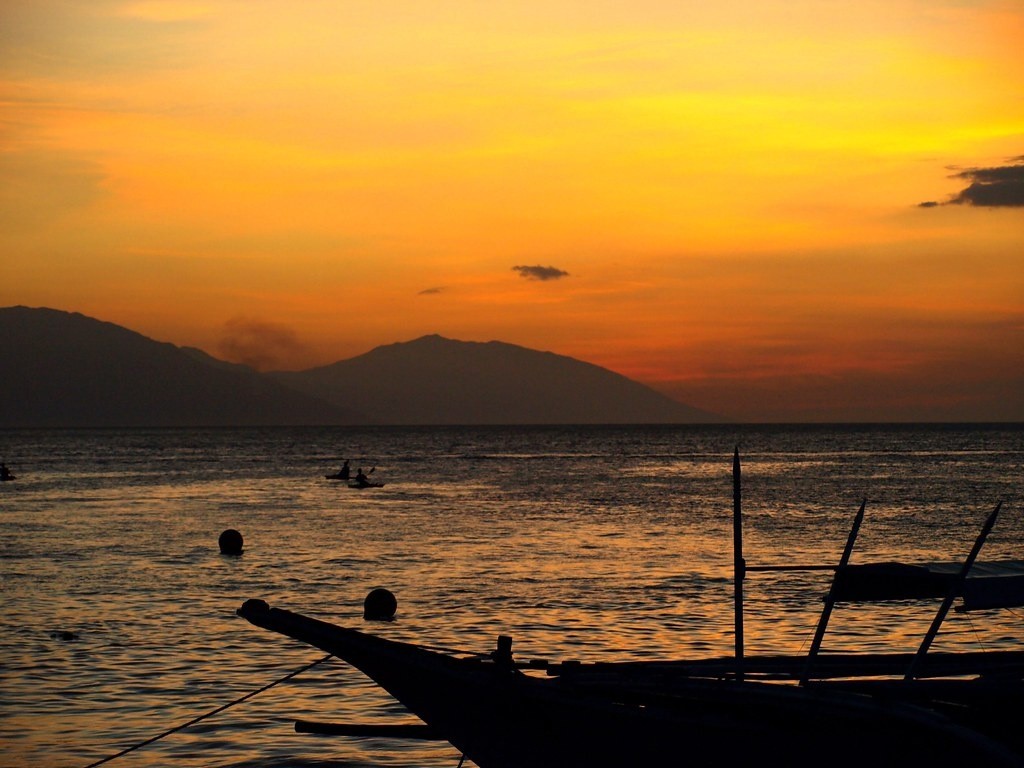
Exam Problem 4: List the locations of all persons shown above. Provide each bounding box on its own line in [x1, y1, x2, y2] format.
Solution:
[337, 461, 350, 478]
[0, 462, 11, 477]
[355, 468, 370, 485]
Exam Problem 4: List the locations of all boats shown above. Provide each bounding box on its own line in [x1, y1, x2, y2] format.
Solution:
[324, 473, 354, 482]
[347, 479, 386, 490]
[231, 446, 1023, 768]
